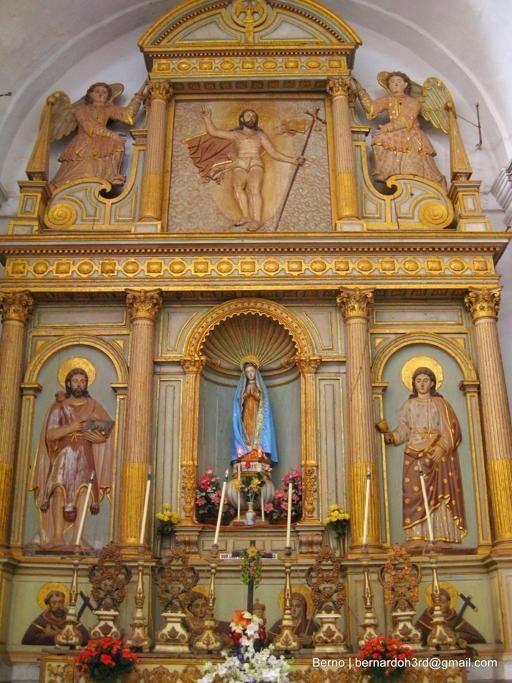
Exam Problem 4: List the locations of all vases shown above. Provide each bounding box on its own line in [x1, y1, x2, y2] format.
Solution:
[245, 502, 257, 525]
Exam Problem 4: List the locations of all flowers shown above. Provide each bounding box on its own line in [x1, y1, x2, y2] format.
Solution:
[156, 502, 181, 536]
[323, 504, 351, 534]
[354, 636, 414, 683]
[195, 465, 237, 525]
[239, 543, 264, 590]
[193, 608, 295, 683]
[263, 464, 305, 524]
[232, 476, 261, 502]
[71, 637, 140, 683]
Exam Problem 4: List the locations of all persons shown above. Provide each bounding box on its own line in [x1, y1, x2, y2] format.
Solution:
[185, 590, 235, 648]
[350, 72, 448, 194]
[198, 104, 306, 233]
[45, 78, 150, 194]
[20, 591, 89, 646]
[384, 367, 468, 542]
[413, 589, 487, 645]
[266, 592, 319, 647]
[42, 368, 107, 518]
[230, 360, 279, 465]
